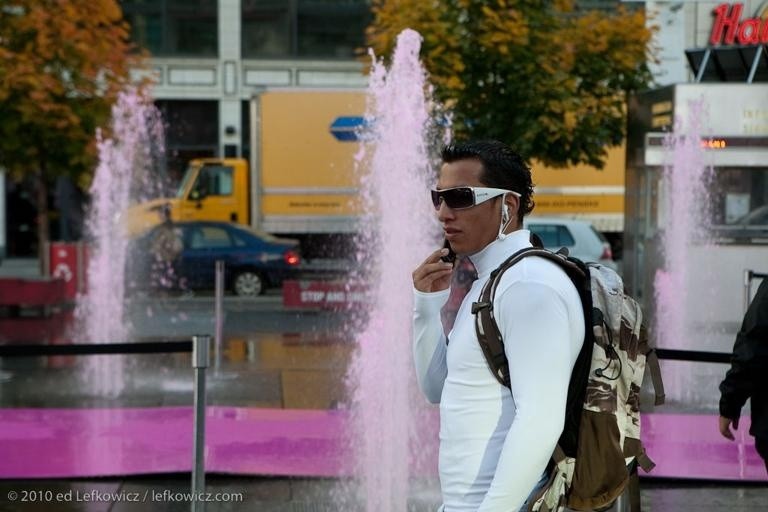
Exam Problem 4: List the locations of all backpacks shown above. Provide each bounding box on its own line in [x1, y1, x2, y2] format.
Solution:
[471, 244, 666, 511]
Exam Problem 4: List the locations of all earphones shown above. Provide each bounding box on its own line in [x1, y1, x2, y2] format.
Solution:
[503, 204, 509, 221]
[499, 224, 505, 240]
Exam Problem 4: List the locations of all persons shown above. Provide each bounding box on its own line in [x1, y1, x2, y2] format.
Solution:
[411, 134, 588, 512]
[717, 272, 768, 477]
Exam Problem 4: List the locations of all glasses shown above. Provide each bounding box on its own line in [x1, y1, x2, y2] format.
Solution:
[430, 186, 522, 211]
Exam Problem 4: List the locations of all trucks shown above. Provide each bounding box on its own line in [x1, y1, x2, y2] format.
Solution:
[124, 89, 627, 262]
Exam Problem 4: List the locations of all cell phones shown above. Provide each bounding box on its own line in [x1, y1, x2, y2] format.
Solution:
[441, 238, 457, 269]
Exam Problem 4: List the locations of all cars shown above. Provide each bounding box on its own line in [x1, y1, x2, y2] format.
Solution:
[124, 220, 302, 297]
[522, 216, 618, 273]
